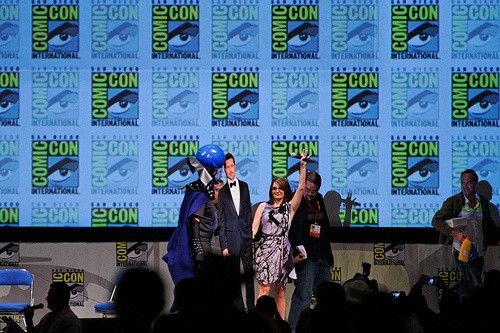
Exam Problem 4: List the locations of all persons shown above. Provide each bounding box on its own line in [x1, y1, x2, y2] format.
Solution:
[163, 144, 225, 314]
[288, 172, 334, 333]
[431, 169, 500, 289]
[1, 263, 500, 333]
[250, 149, 308, 320]
[217, 152, 258, 311]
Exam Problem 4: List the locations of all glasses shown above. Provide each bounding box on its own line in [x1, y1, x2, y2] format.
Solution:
[272, 187, 284, 190]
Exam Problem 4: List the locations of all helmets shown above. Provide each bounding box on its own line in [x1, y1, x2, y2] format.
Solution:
[195, 144, 226, 168]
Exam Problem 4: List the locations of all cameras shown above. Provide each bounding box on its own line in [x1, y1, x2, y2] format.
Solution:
[428, 277, 437, 284]
[391, 292, 400, 297]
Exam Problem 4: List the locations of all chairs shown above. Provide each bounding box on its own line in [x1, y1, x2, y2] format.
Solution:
[94, 271, 124, 318]
[0, 268, 35, 333]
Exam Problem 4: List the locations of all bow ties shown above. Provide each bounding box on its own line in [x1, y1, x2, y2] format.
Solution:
[230, 181, 237, 188]
